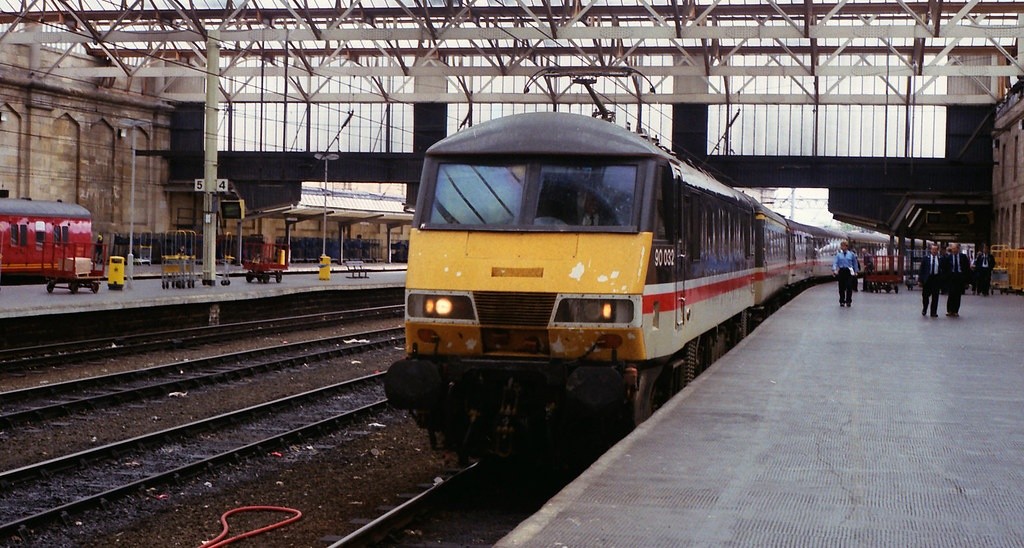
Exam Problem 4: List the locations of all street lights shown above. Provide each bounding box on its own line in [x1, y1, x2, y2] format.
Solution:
[117, 118, 153, 290]
[315, 153, 339, 255]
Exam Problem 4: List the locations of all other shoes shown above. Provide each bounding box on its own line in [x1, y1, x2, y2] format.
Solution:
[945, 311, 952, 316]
[922, 309, 928, 316]
[846, 302, 851, 307]
[840, 302, 846, 307]
[952, 312, 960, 317]
[930, 313, 939, 318]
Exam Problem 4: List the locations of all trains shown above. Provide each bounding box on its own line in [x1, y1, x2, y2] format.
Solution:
[0, 197, 94, 285]
[404, 64, 975, 433]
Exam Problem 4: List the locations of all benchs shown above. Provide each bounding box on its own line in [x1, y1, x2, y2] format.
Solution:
[345, 260, 371, 279]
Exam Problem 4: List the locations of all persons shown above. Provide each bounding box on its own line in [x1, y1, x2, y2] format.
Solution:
[918, 243, 996, 317]
[832, 241, 860, 306]
[577, 194, 613, 226]
[860, 248, 875, 291]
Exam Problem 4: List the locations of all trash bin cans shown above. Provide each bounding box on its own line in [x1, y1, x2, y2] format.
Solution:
[318, 257, 330, 279]
[107, 256, 125, 289]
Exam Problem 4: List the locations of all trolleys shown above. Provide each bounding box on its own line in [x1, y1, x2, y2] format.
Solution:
[161, 230, 196, 289]
[221, 232, 233, 286]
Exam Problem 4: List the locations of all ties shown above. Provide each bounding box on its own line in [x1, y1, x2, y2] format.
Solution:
[954, 254, 963, 274]
[932, 255, 935, 276]
[984, 253, 988, 265]
